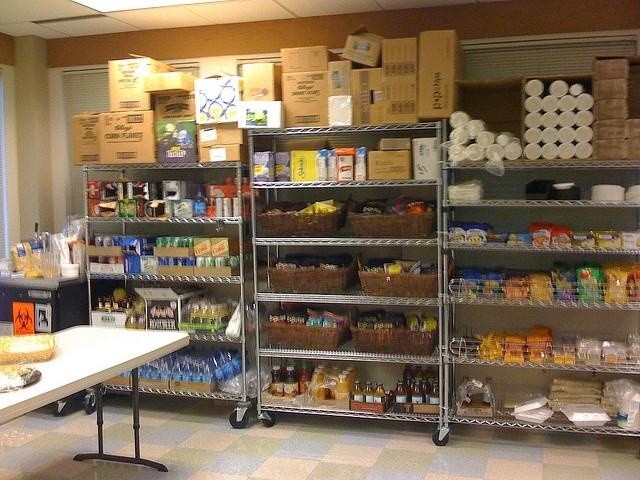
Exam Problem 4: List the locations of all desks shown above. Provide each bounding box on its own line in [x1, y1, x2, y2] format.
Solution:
[0, 322, 191, 472]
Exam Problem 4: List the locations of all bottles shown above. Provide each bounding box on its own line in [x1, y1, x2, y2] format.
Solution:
[268, 359, 441, 414]
[224, 175, 248, 185]
[206, 192, 216, 217]
[480, 376, 495, 406]
[460, 375, 472, 407]
[192, 191, 205, 217]
[94, 291, 239, 335]
[32, 221, 40, 249]
[120, 344, 250, 385]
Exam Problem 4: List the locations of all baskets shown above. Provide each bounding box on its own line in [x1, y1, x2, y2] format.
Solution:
[266, 255, 356, 292]
[0, 332, 57, 364]
[258, 198, 348, 239]
[262, 309, 349, 350]
[357, 254, 438, 297]
[346, 198, 437, 239]
[348, 312, 439, 356]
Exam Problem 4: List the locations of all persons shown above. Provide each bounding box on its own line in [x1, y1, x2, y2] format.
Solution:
[38, 310, 49, 327]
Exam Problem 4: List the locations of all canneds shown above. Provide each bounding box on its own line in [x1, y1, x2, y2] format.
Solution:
[95, 236, 239, 267]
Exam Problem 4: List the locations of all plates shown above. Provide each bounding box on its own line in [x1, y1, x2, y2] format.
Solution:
[626, 185, 640, 203]
[552, 181, 576, 191]
[590, 183, 625, 202]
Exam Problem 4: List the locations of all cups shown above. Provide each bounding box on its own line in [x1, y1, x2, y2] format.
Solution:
[32, 250, 62, 280]
